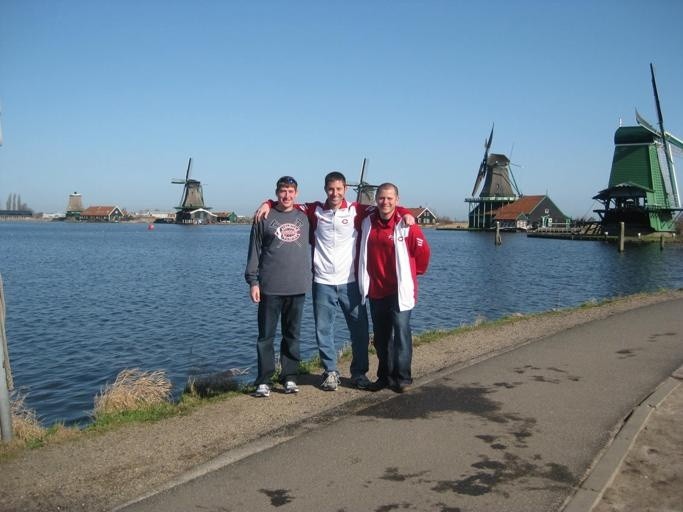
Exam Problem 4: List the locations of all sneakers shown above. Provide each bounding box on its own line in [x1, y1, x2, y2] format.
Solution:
[320, 371, 340, 391]
[255, 382, 274, 397]
[282, 380, 299, 394]
[367, 375, 395, 392]
[350, 374, 372, 390]
[393, 379, 417, 393]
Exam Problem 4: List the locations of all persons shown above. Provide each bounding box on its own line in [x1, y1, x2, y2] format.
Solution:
[353, 184, 429, 393]
[253, 171, 416, 391]
[242, 175, 311, 397]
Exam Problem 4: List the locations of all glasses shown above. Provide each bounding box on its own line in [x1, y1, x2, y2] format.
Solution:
[277, 178, 297, 185]
